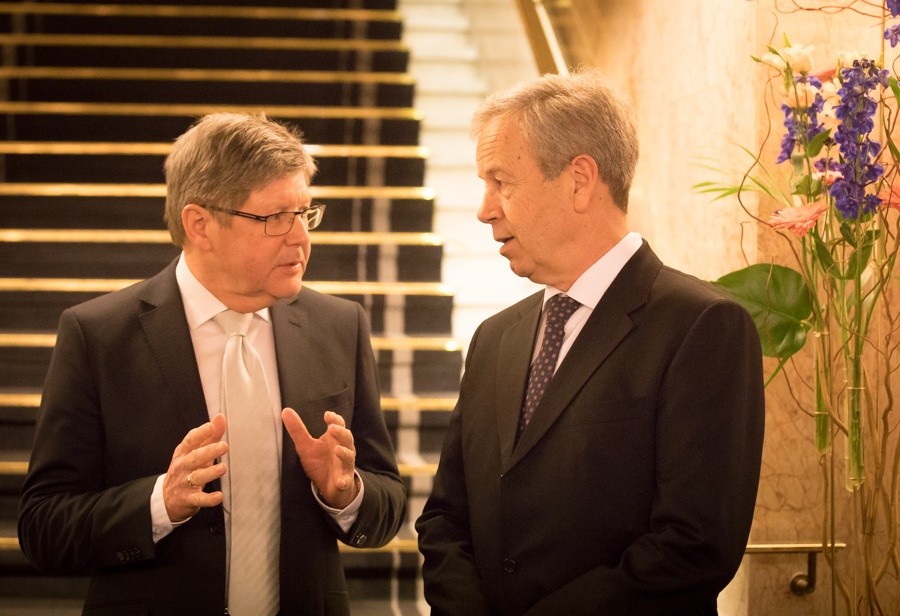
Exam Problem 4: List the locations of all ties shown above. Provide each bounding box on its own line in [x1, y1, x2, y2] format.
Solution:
[515, 295, 582, 446]
[214, 310, 282, 616]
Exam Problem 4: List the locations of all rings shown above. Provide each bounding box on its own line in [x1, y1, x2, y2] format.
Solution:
[187, 473, 194, 486]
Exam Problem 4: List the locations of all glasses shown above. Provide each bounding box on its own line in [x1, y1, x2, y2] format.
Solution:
[199, 202, 326, 236]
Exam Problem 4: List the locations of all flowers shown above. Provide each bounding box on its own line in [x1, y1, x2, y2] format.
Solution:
[691, 0, 900, 616]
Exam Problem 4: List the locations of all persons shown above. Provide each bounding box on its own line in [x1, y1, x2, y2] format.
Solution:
[415, 70, 767, 616]
[16, 113, 407, 616]
